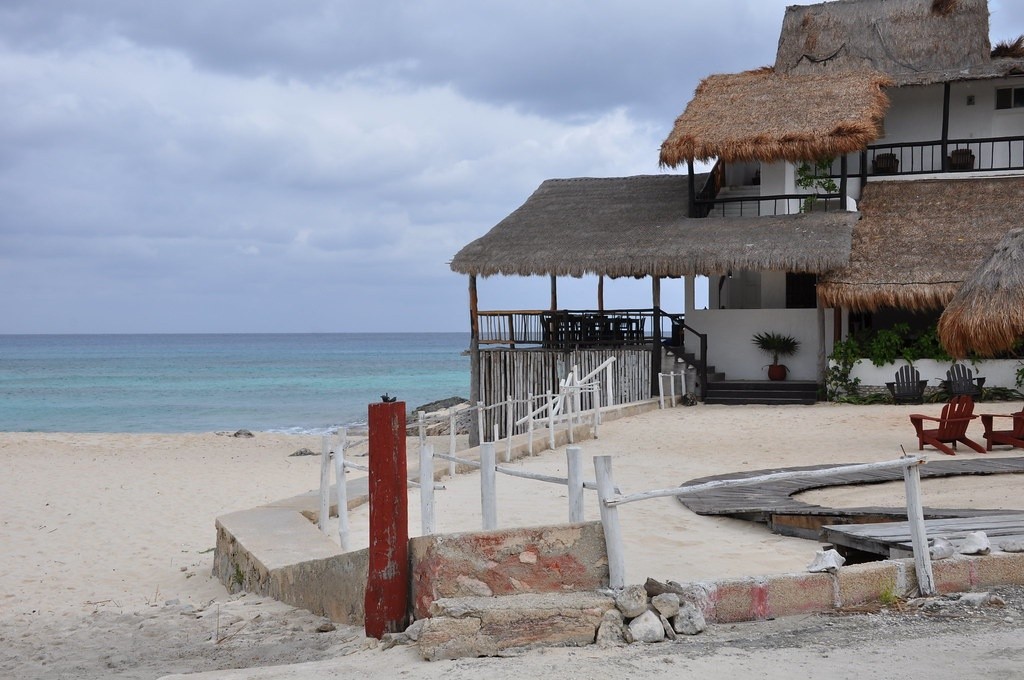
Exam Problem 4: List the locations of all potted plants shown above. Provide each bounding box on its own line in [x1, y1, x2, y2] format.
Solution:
[752, 332, 802, 382]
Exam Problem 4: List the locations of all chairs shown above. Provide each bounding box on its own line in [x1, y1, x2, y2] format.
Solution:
[910, 394, 988, 456]
[939, 364, 986, 404]
[872, 152, 899, 175]
[947, 149, 973, 170]
[885, 365, 929, 405]
[539, 309, 646, 349]
[980, 406, 1024, 452]
